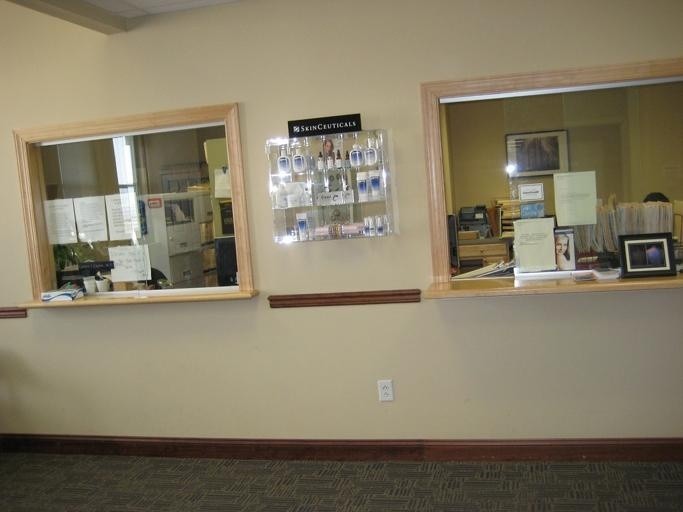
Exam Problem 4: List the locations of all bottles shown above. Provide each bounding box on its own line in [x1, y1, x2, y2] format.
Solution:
[317, 150, 350, 168]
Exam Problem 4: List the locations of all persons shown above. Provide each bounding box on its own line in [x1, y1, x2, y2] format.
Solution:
[554, 232, 574, 270]
[317, 139, 336, 163]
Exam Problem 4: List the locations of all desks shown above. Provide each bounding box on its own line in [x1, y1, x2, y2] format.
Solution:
[457, 240, 510, 273]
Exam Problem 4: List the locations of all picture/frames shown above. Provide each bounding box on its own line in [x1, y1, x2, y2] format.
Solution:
[505, 129, 570, 181]
[616, 231, 677, 279]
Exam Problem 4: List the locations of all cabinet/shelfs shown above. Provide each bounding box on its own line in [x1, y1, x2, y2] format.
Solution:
[265, 124, 398, 247]
[143, 193, 204, 289]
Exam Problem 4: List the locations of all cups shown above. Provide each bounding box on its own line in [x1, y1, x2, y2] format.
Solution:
[96, 277, 113, 292]
[84, 276, 97, 293]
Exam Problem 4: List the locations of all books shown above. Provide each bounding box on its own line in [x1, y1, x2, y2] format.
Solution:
[486, 208, 502, 237]
[568, 192, 674, 254]
[450, 262, 500, 279]
[496, 199, 521, 240]
